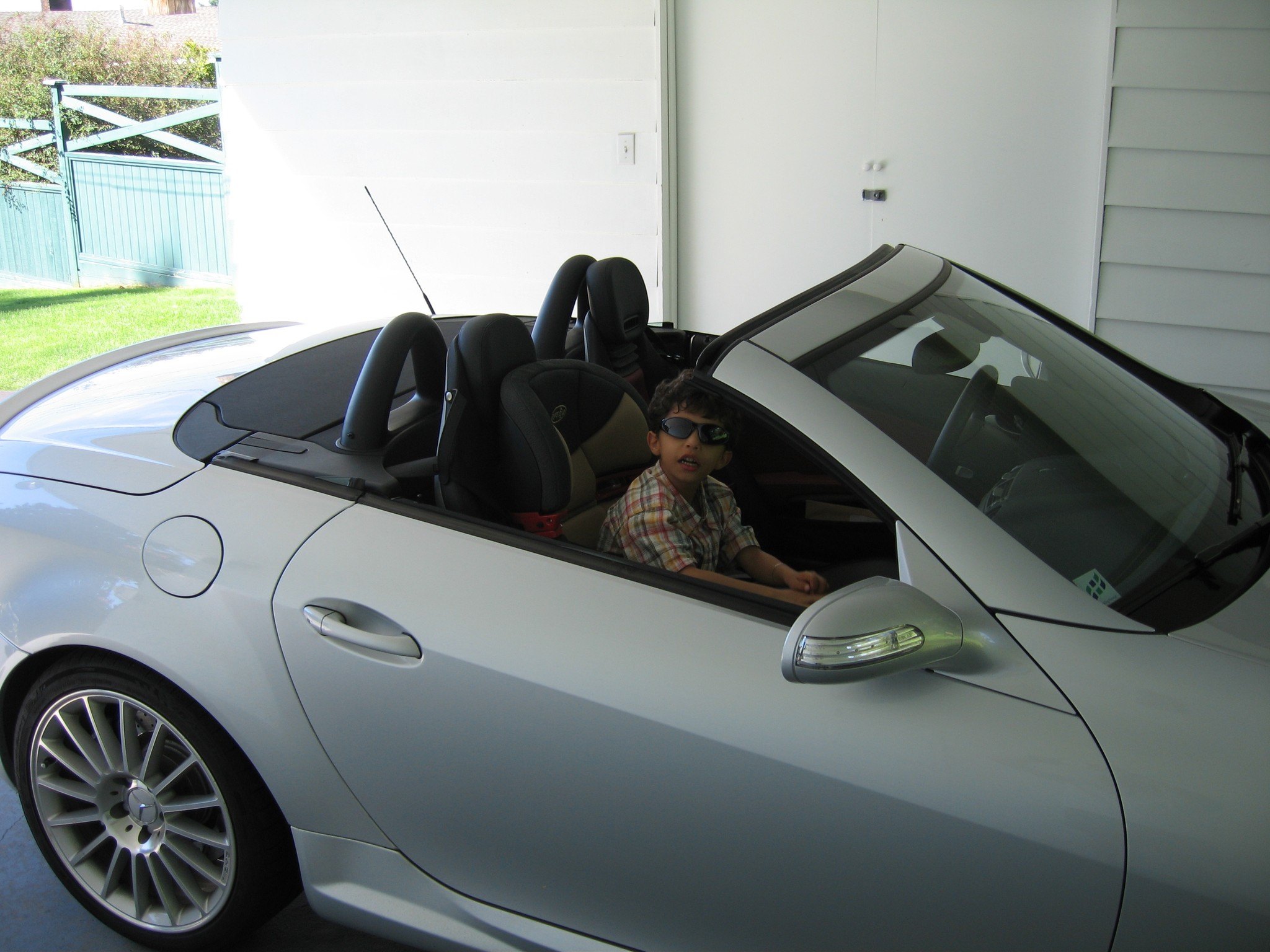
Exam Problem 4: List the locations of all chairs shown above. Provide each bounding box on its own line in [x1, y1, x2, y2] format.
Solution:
[434, 256, 659, 549]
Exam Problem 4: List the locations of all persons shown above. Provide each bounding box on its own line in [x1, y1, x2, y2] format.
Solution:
[596, 368, 829, 605]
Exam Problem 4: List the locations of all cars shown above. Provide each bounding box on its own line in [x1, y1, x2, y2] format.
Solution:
[0, 240, 1270, 952]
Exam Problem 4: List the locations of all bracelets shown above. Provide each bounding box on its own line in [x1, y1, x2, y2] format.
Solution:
[771, 563, 786, 584]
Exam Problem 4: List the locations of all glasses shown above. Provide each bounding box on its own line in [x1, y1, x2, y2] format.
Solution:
[651, 417, 728, 449]
